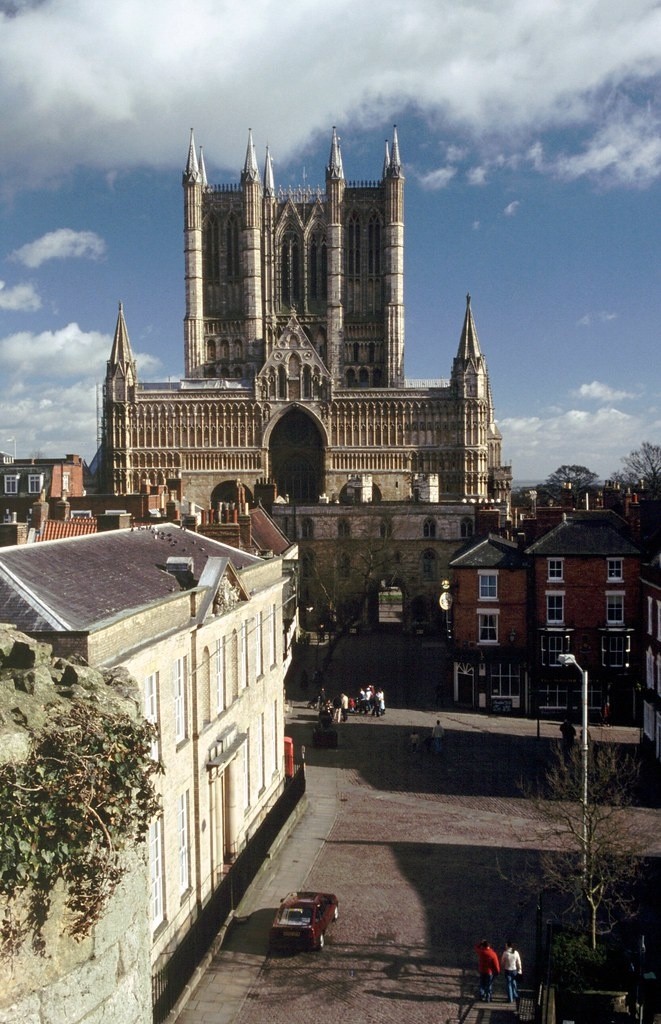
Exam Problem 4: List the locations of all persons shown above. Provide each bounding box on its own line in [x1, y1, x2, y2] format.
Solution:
[502, 944, 522, 1002]
[474, 937, 501, 1003]
[316, 684, 385, 723]
[559, 717, 576, 758]
[433, 719, 444, 752]
[409, 731, 420, 753]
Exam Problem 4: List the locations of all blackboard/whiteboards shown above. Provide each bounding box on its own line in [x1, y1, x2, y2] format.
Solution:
[492, 698, 512, 714]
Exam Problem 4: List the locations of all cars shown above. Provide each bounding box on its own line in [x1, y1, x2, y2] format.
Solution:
[268, 890, 339, 950]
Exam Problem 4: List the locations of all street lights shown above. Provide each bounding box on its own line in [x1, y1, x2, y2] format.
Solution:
[559, 654, 587, 877]
